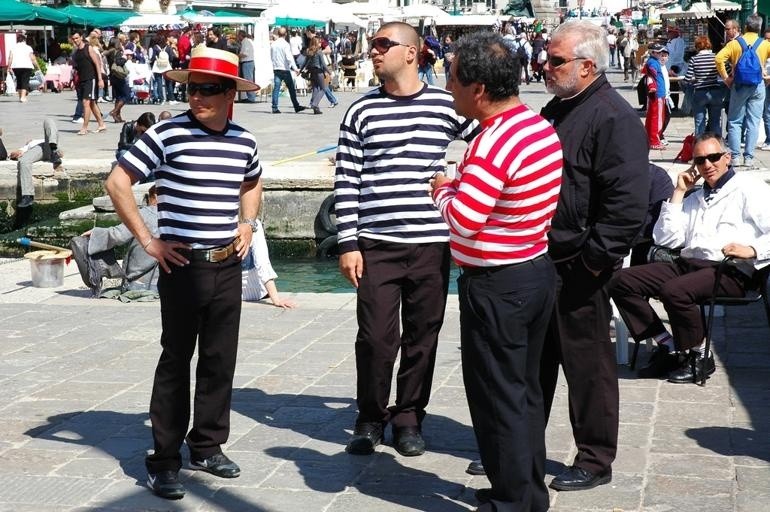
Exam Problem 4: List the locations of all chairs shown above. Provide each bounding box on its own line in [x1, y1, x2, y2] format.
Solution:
[272, 60, 374, 106]
[29, 60, 188, 105]
[626, 244, 770, 389]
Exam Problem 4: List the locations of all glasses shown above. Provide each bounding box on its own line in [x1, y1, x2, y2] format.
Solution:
[186, 82, 230, 96]
[546, 53, 597, 69]
[693, 152, 727, 165]
[367, 37, 410, 55]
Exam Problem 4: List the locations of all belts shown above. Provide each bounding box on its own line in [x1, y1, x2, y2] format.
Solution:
[173, 238, 241, 264]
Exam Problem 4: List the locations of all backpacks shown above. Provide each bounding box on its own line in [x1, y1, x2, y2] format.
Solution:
[154, 45, 169, 67]
[516, 41, 528, 65]
[734, 35, 765, 90]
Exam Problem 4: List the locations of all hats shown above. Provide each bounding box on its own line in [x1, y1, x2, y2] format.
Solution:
[648, 43, 669, 56]
[163, 46, 260, 92]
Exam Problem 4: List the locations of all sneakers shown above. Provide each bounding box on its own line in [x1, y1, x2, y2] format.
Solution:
[345, 421, 386, 455]
[147, 470, 185, 497]
[188, 452, 240, 478]
[271, 100, 338, 115]
[54, 154, 62, 164]
[72, 111, 125, 135]
[649, 138, 668, 150]
[18, 195, 34, 208]
[393, 430, 426, 456]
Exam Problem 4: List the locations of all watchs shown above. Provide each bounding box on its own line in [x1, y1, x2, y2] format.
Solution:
[238, 218, 259, 232]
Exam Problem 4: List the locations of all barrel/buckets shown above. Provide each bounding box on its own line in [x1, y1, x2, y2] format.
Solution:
[24, 250, 72, 288]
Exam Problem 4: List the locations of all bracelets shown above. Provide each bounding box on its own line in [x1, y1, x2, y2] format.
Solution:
[142, 234, 153, 250]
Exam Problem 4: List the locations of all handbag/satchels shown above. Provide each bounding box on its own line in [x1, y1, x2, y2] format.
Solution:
[111, 62, 130, 80]
[537, 50, 548, 65]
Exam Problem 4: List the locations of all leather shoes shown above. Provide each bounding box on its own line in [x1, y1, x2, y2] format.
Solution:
[550, 466, 612, 491]
[637, 344, 689, 379]
[668, 350, 716, 384]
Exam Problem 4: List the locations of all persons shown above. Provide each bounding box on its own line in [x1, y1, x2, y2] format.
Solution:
[333, 21, 480, 458]
[466, 19, 650, 490]
[611, 132, 769, 384]
[103, 46, 262, 499]
[79, 184, 163, 300]
[241, 219, 293, 310]
[428, 33, 565, 511]
